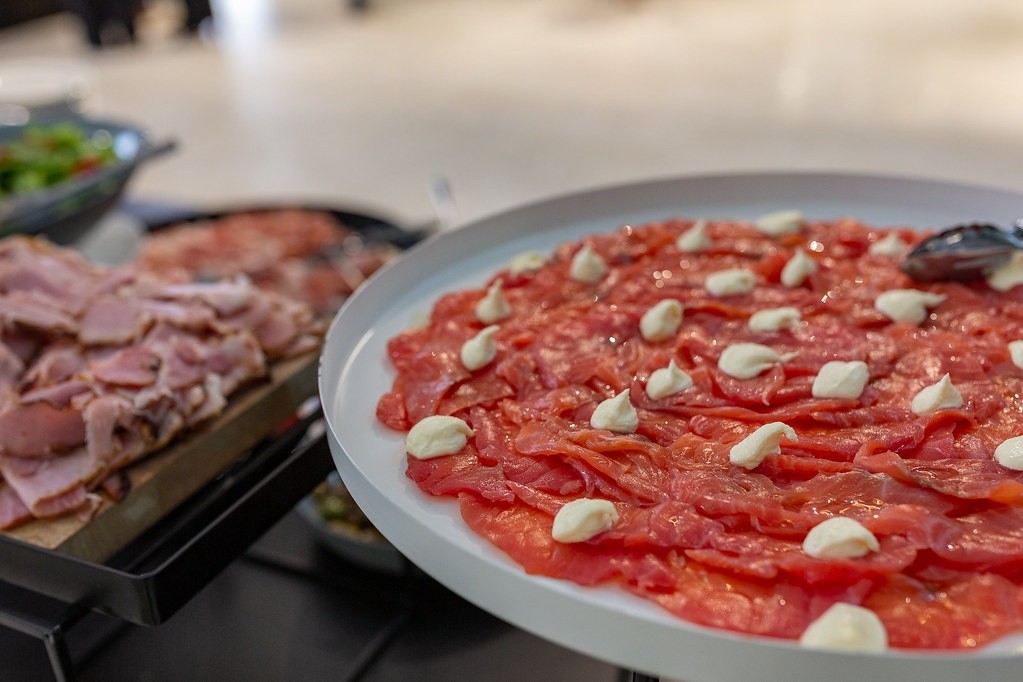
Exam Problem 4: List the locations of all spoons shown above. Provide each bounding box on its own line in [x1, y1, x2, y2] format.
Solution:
[901, 225, 1022, 281]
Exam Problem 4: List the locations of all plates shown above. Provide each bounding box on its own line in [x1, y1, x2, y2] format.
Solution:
[1, 102, 181, 234]
[1, 203, 436, 629]
[317, 169, 1023, 682]
